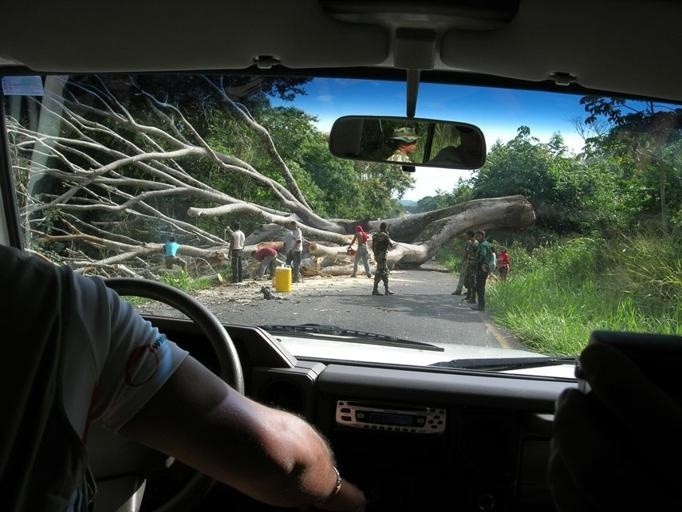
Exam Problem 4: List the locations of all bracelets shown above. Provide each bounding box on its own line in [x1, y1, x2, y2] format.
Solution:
[332, 467, 342, 496]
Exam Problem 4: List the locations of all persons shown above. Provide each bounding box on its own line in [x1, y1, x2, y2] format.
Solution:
[470, 229, 492, 310]
[250, 248, 278, 281]
[2, 241, 368, 512]
[349, 225, 372, 278]
[282, 223, 293, 254]
[498, 245, 511, 284]
[370, 222, 397, 296]
[163, 236, 188, 275]
[225, 222, 246, 284]
[451, 230, 479, 300]
[430, 124, 483, 166]
[383, 126, 422, 164]
[489, 247, 499, 282]
[287, 220, 303, 285]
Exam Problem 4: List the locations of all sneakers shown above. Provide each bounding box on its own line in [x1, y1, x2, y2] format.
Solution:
[450, 290, 486, 311]
[350, 273, 372, 278]
[371, 291, 395, 296]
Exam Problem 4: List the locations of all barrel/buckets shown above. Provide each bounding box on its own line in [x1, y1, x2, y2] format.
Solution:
[275, 263, 292, 291]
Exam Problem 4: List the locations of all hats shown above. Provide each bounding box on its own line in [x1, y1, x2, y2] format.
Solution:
[390, 127, 423, 145]
